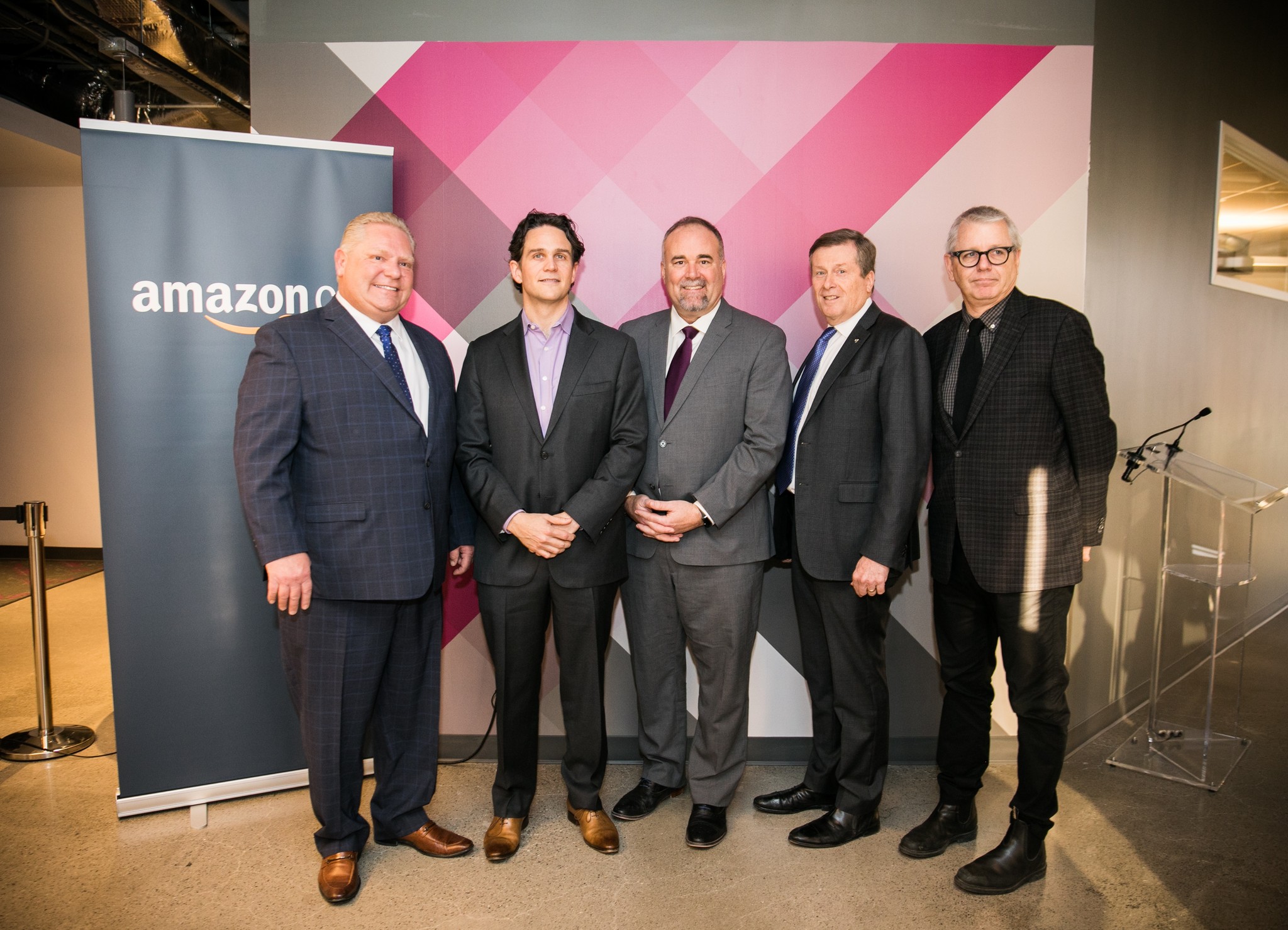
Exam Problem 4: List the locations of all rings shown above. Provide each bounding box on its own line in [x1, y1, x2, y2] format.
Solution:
[867, 587, 876, 593]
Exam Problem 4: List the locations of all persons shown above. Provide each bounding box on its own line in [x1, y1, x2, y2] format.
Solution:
[748, 228, 936, 848]
[895, 204, 1121, 899]
[229, 208, 477, 904]
[599, 213, 793, 851]
[451, 204, 651, 865]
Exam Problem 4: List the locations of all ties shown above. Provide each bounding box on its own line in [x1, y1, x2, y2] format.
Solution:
[952, 318, 986, 442]
[664, 326, 699, 423]
[375, 325, 414, 412]
[773, 326, 836, 496]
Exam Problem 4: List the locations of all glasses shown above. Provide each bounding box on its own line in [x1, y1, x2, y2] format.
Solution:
[947, 245, 1016, 268]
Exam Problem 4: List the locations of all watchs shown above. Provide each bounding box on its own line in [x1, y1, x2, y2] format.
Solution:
[696, 506, 713, 528]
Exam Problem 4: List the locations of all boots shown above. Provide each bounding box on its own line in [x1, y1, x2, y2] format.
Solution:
[954, 805, 1047, 894]
[898, 796, 978, 857]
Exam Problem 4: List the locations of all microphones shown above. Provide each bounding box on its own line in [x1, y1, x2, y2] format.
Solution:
[1126, 407, 1212, 462]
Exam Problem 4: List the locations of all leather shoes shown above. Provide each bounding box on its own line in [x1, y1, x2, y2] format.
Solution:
[567, 796, 620, 853]
[788, 802, 881, 849]
[374, 819, 475, 858]
[685, 802, 728, 848]
[753, 782, 837, 814]
[318, 851, 362, 902]
[611, 777, 688, 821]
[484, 812, 529, 861]
